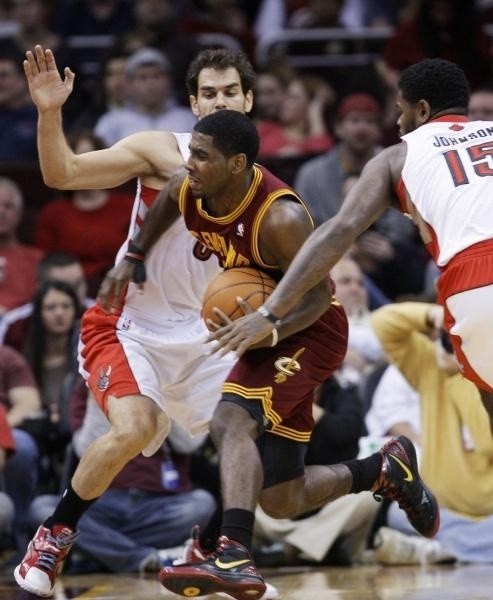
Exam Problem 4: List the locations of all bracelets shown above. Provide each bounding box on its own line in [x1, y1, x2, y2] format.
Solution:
[124, 251, 145, 262]
[257, 304, 282, 325]
[271, 328, 278, 347]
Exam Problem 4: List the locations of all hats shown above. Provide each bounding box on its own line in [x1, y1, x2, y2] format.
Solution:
[126, 47, 170, 75]
[338, 93, 379, 119]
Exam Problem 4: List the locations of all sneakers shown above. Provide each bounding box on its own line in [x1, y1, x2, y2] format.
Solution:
[155, 545, 184, 568]
[159, 537, 266, 600]
[374, 527, 439, 567]
[172, 524, 278, 600]
[372, 434, 440, 537]
[12, 523, 81, 597]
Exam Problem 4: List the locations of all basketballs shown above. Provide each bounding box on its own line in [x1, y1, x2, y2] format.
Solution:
[202, 267, 278, 333]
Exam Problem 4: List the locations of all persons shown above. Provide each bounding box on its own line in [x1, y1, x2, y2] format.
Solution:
[0, 0, 68, 57]
[99, 110, 443, 600]
[0, 178, 43, 312]
[12, 45, 254, 596]
[370, 302, 493, 563]
[94, 48, 197, 148]
[0, 252, 95, 351]
[250, 70, 285, 135]
[0, 35, 69, 170]
[21, 282, 84, 440]
[259, 76, 333, 158]
[202, 57, 493, 392]
[0, 345, 42, 522]
[37, 129, 135, 299]
[296, 94, 417, 273]
[87, 51, 134, 124]
[467, 90, 492, 122]
[26, 443, 217, 575]
[327, 259, 390, 413]
[364, 361, 421, 467]
